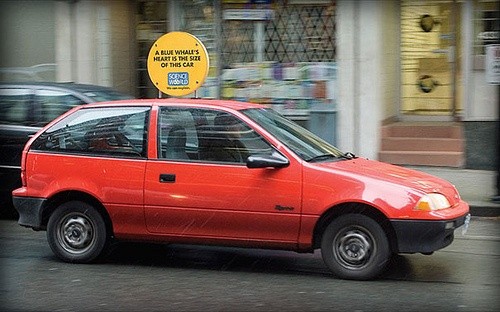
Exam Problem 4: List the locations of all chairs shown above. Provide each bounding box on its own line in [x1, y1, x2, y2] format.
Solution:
[166, 127, 191, 160]
[206, 112, 229, 161]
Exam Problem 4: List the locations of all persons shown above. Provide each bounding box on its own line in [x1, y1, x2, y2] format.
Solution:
[206, 112, 252, 163]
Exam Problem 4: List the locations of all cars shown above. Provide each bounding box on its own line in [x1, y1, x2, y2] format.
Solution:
[12, 99, 471, 280]
[0, 83, 135, 186]
